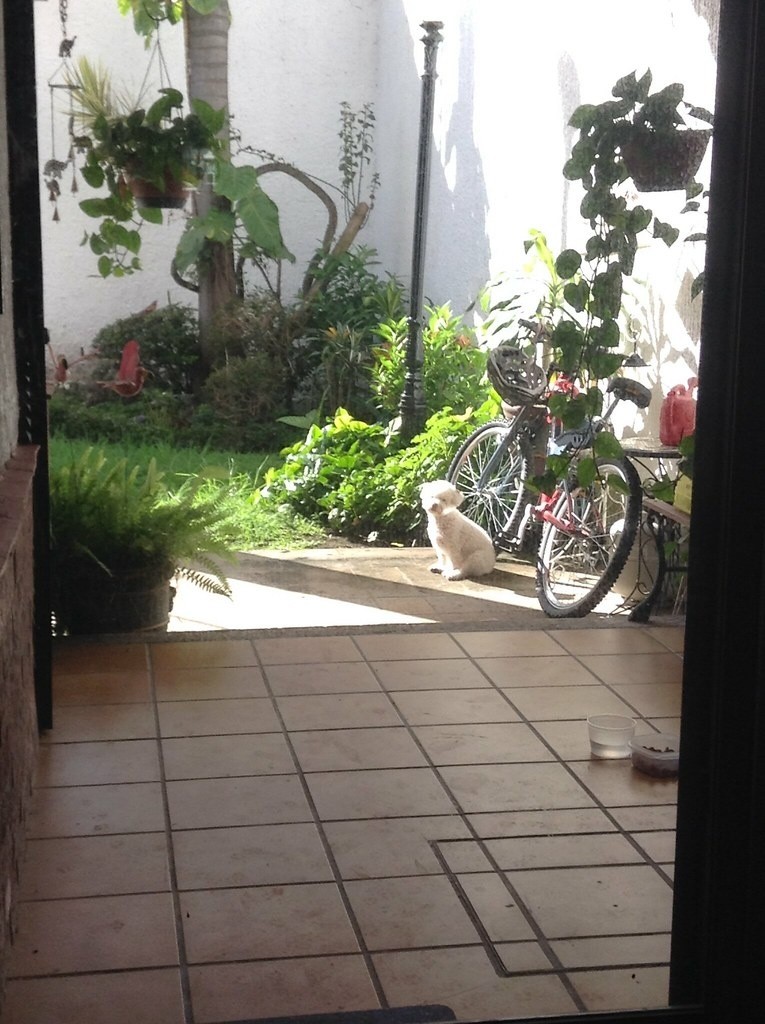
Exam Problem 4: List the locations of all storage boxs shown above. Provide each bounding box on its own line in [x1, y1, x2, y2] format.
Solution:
[627, 734, 679, 777]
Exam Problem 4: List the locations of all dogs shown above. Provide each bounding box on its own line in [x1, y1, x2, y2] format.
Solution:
[416, 479, 496, 582]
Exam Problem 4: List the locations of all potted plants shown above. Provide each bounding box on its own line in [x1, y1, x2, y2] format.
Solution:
[522, 66, 713, 503]
[47, 445, 241, 632]
[66, 89, 230, 279]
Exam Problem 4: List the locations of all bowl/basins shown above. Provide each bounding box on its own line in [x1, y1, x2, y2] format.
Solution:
[587, 713, 637, 759]
[627, 733, 679, 777]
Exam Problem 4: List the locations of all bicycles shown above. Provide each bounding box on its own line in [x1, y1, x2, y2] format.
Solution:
[443, 318, 644, 620]
